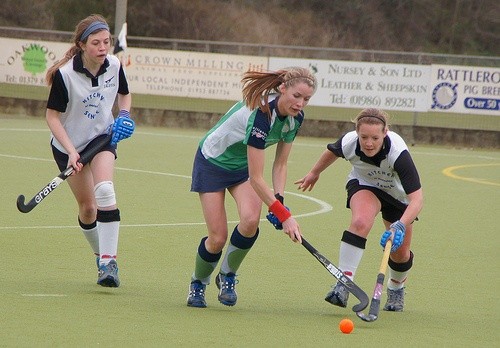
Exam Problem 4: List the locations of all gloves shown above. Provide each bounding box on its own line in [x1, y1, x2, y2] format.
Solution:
[266, 194, 289, 230]
[109, 110, 135, 146]
[381, 221, 406, 252]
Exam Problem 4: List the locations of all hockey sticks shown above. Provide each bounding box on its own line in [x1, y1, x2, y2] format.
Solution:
[16, 135, 112, 214]
[294, 234, 369, 312]
[356, 228, 396, 322]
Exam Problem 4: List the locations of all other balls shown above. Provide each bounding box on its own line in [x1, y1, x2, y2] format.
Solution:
[339, 319, 354, 334]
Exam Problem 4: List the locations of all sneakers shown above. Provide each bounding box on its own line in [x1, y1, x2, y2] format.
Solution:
[325, 282, 351, 308]
[383, 286, 407, 311]
[186, 280, 207, 308]
[215, 272, 240, 307]
[94, 254, 121, 288]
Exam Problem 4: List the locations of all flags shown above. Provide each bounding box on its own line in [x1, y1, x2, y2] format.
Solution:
[113, 23, 128, 54]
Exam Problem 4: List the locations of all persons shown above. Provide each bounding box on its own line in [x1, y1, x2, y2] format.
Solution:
[186, 67, 317, 309]
[294, 107, 424, 312]
[45, 15, 135, 286]
